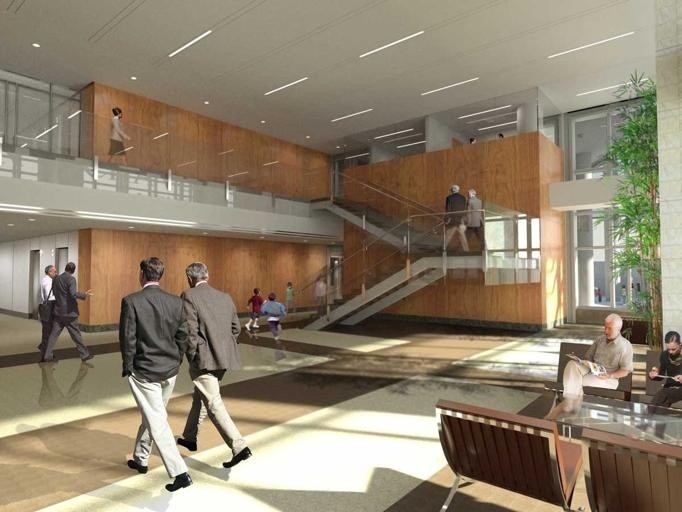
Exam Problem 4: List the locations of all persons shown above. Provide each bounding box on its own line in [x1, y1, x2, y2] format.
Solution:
[285, 282, 297, 316]
[109, 108, 131, 170]
[38, 265, 58, 360]
[595, 281, 640, 303]
[179, 261, 253, 470]
[244, 287, 265, 330]
[442, 185, 470, 254]
[315, 275, 326, 318]
[41, 262, 95, 362]
[117, 256, 194, 492]
[561, 312, 634, 416]
[262, 293, 286, 341]
[648, 330, 682, 408]
[458, 189, 484, 252]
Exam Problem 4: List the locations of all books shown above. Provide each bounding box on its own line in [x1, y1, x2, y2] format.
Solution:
[565, 352, 605, 376]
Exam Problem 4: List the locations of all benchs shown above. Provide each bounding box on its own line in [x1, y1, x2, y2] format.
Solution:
[580, 427, 681, 511]
[435, 398, 587, 511]
[638, 350, 681, 409]
[544, 341, 632, 406]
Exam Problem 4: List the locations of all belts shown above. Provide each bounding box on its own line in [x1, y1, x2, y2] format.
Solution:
[43, 301, 56, 303]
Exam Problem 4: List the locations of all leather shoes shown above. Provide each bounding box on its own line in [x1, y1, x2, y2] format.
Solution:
[178, 438, 197, 451]
[223, 447, 252, 468]
[128, 460, 147, 473]
[39, 344, 56, 361]
[166, 472, 193, 491]
[82, 356, 94, 361]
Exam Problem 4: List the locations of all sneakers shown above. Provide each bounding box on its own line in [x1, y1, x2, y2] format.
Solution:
[253, 324, 259, 328]
[245, 324, 251, 331]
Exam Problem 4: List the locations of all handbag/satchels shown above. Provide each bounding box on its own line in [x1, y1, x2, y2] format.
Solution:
[39, 303, 52, 322]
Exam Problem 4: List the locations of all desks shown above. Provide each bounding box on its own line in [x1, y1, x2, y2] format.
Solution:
[543, 394, 682, 461]
